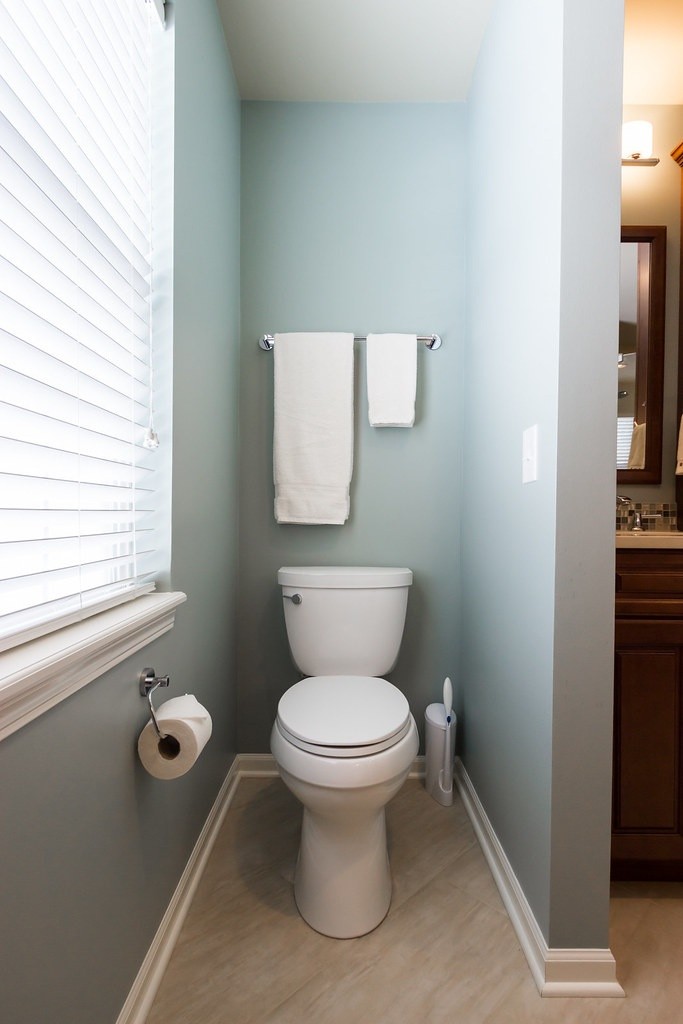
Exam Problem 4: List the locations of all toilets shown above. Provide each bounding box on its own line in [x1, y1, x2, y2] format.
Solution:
[270, 566, 420, 940]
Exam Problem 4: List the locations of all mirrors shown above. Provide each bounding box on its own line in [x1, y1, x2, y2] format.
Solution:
[617, 222, 666, 488]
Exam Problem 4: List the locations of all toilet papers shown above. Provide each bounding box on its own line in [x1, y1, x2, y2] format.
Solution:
[138, 693, 213, 779]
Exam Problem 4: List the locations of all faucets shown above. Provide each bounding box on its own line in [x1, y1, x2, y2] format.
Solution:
[616, 493, 633, 512]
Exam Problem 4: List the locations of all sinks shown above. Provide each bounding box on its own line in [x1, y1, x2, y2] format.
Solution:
[616, 533, 636, 536]
[638, 533, 683, 536]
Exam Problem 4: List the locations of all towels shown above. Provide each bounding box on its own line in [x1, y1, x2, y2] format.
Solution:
[273, 332, 355, 525]
[628, 422, 647, 470]
[675, 413, 683, 475]
[366, 332, 418, 428]
